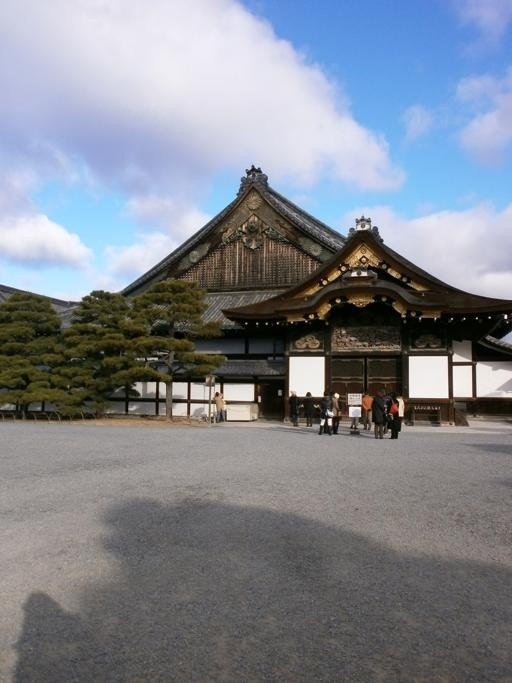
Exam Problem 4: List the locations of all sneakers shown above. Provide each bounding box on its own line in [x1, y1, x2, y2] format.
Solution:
[318, 425, 339, 435]
[350, 425, 401, 440]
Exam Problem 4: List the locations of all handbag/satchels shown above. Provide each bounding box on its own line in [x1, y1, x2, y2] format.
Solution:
[390, 403, 399, 415]
[325, 409, 335, 419]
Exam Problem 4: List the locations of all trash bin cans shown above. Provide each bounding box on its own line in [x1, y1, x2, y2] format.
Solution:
[455, 401, 467, 426]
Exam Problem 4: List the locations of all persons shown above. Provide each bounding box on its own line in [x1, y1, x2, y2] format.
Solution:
[288, 389, 408, 439]
[214, 392, 228, 422]
